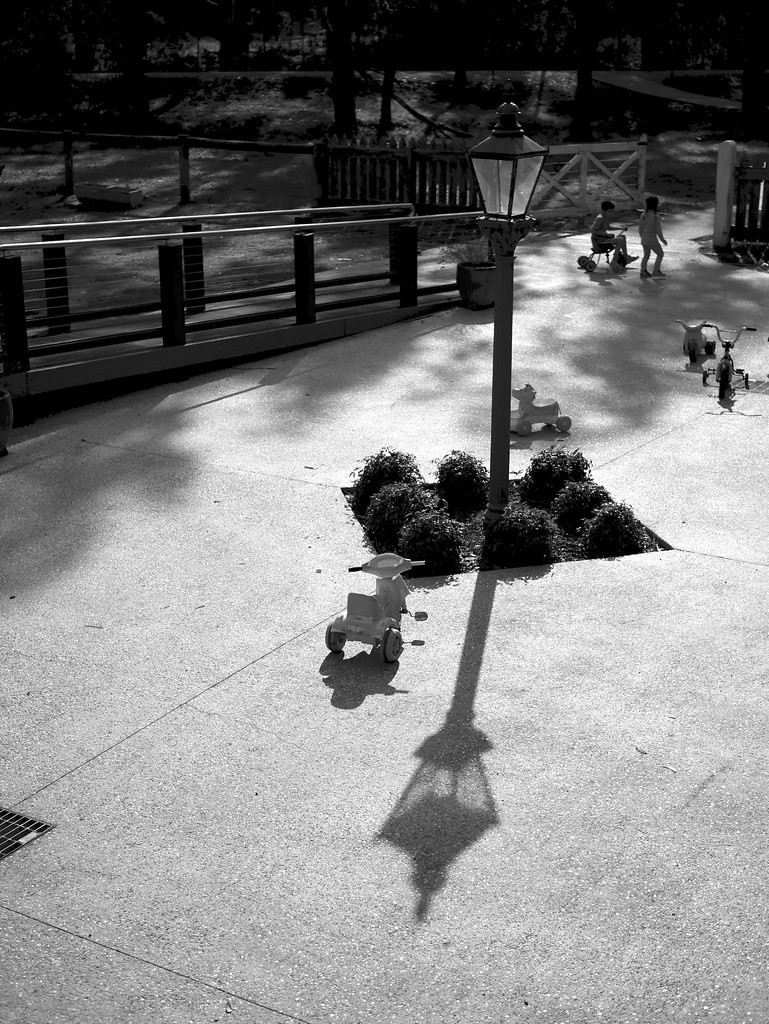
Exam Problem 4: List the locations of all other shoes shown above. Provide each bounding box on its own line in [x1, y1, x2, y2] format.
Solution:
[624, 255, 639, 265]
[609, 261, 625, 270]
[650, 270, 665, 277]
[640, 271, 652, 278]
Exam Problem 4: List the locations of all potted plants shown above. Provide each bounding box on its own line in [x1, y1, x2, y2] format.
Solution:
[437, 241, 495, 312]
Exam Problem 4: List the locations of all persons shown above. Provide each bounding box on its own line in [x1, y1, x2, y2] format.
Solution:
[589, 201, 639, 271]
[639, 196, 668, 278]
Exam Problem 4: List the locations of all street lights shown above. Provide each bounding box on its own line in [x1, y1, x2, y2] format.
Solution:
[461, 75, 552, 524]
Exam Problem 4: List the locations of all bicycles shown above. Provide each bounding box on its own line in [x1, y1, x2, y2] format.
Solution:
[701, 321, 757, 404]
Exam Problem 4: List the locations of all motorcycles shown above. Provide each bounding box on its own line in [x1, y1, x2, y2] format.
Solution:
[675, 319, 716, 363]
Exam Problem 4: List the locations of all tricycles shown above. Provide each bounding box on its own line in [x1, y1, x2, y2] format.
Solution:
[577, 226, 630, 273]
[322, 553, 429, 662]
